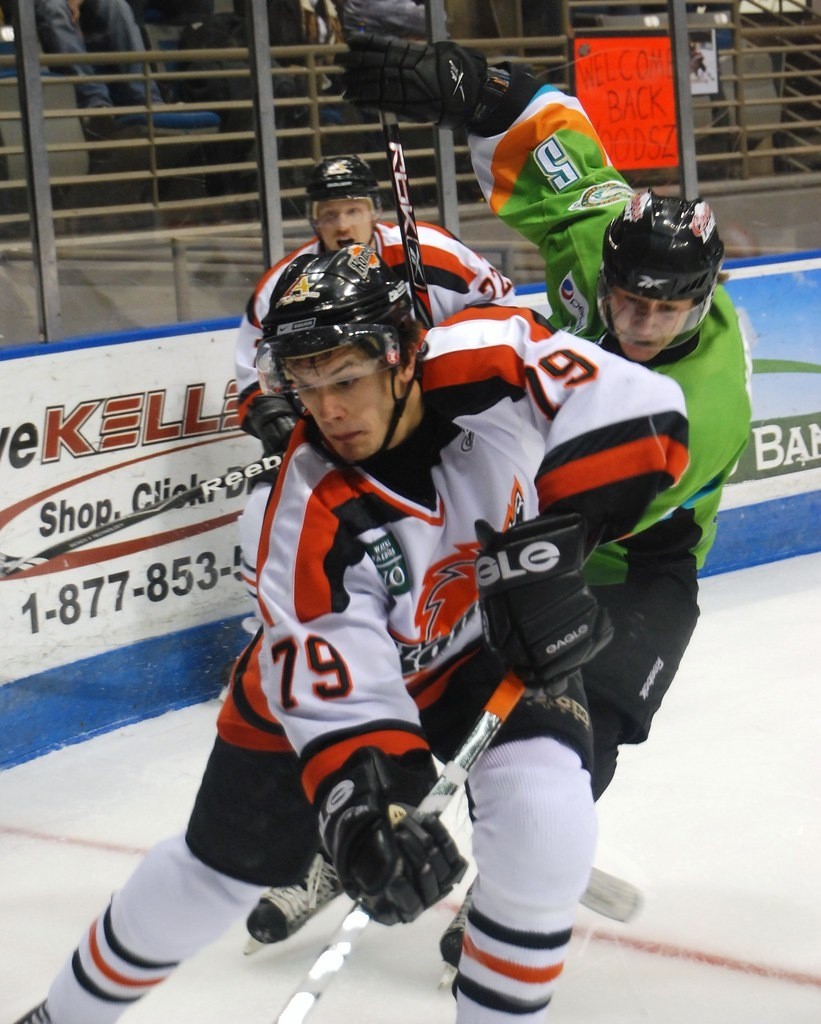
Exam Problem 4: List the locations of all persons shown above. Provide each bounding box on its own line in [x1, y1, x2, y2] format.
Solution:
[243, 28, 753, 975]
[9, 239, 694, 1024]
[1, 0, 444, 217]
[233, 150, 520, 473]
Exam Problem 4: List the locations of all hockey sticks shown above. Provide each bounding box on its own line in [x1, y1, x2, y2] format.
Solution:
[366, 104, 644, 922]
[269, 677, 529, 1024]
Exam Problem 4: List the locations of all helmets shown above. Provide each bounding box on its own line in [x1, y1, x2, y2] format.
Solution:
[596, 188, 724, 350]
[255, 242, 414, 394]
[304, 156, 382, 228]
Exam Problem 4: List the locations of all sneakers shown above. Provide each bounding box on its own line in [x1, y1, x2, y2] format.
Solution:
[245, 855, 344, 958]
[438, 873, 478, 987]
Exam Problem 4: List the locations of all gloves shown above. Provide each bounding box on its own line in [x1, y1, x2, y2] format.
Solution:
[315, 745, 468, 924]
[474, 514, 614, 702]
[246, 400, 297, 479]
[334, 35, 488, 130]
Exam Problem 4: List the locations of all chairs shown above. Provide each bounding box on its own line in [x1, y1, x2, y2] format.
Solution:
[0, 2, 820, 277]
